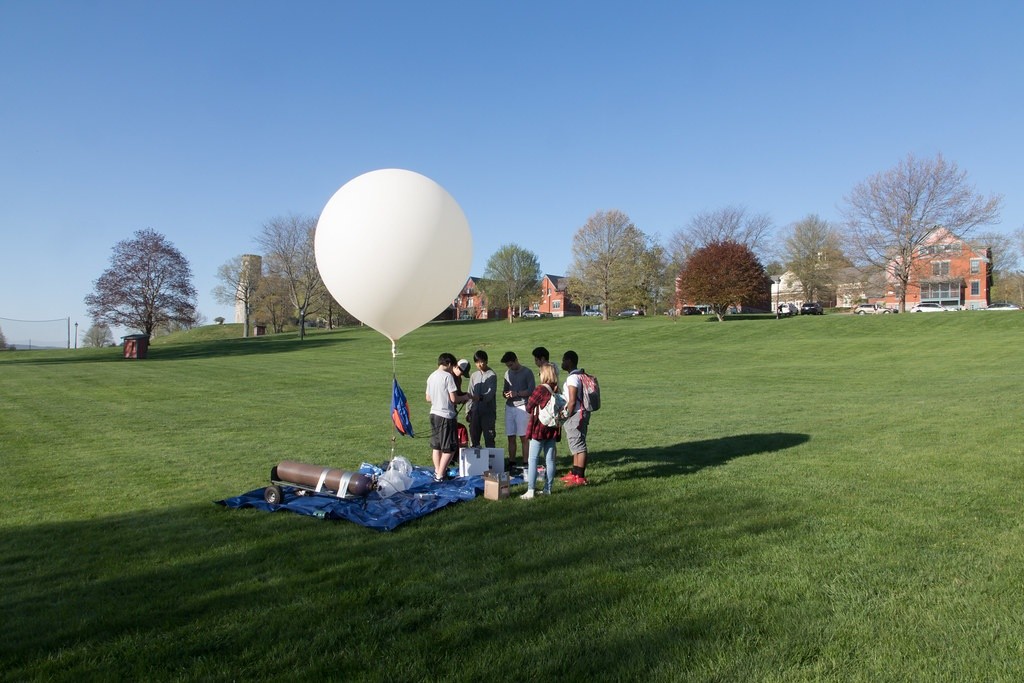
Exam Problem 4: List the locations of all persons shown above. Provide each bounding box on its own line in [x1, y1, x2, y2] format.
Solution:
[560, 350, 590, 487]
[531, 347, 558, 376]
[452, 358, 470, 396]
[778, 304, 785, 314]
[466, 350, 497, 448]
[426, 353, 469, 482]
[501, 351, 535, 467]
[520, 364, 562, 499]
[872, 302, 879, 315]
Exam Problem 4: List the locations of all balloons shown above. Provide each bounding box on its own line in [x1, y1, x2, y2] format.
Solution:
[314, 169, 472, 357]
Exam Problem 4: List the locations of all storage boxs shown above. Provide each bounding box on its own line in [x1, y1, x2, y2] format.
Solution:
[483, 470, 511, 501]
[458, 446, 504, 478]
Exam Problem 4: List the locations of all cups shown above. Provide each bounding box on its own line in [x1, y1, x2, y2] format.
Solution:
[523, 468, 528, 482]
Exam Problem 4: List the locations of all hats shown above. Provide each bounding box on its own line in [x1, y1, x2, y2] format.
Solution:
[457, 359, 471, 378]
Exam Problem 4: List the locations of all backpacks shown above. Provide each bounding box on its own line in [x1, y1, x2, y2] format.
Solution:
[538, 384, 570, 428]
[570, 369, 601, 412]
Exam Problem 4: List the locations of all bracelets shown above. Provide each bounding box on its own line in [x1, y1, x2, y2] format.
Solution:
[517, 391, 520, 396]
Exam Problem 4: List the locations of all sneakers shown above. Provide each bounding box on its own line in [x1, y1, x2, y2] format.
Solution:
[565, 474, 587, 486]
[560, 470, 575, 482]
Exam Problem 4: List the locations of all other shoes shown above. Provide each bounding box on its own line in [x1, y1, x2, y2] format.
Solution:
[520, 494, 534, 500]
[535, 491, 551, 495]
[435, 474, 455, 482]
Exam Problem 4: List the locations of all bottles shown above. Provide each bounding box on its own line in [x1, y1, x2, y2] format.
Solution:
[413, 493, 441, 499]
[312, 510, 330, 519]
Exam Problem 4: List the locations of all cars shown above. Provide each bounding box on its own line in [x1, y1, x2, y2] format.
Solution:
[581, 308, 604, 317]
[777, 303, 798, 317]
[616, 308, 644, 317]
[909, 302, 951, 312]
[522, 309, 554, 319]
[977, 302, 1023, 310]
[854, 303, 894, 315]
[679, 307, 702, 316]
[801, 302, 824, 315]
